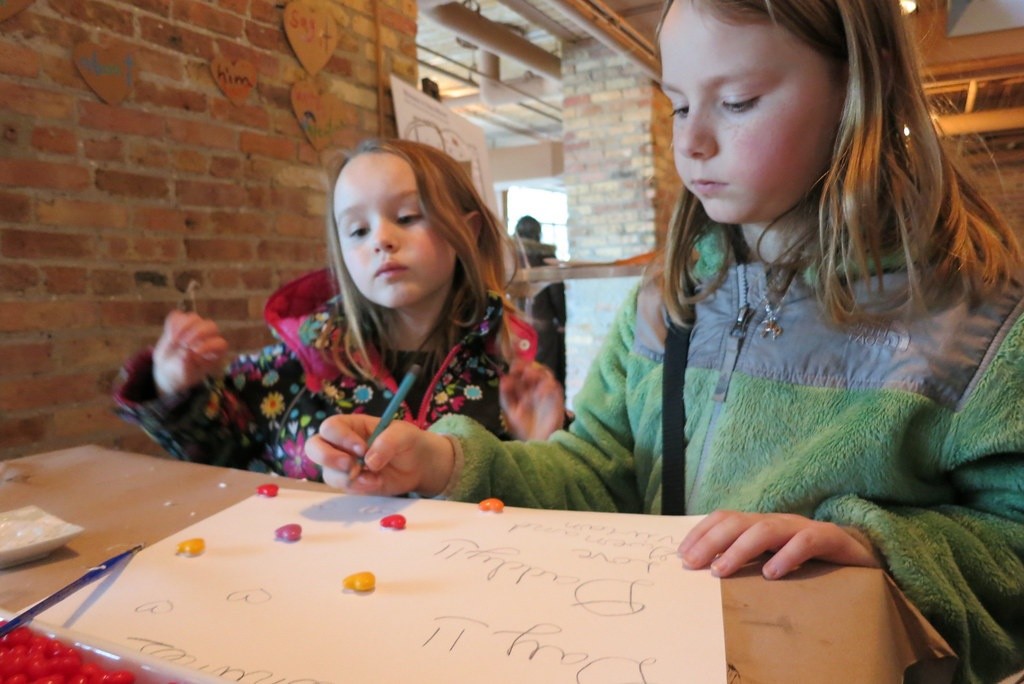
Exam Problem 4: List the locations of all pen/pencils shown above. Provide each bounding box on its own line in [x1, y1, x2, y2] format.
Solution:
[349, 364, 422, 481]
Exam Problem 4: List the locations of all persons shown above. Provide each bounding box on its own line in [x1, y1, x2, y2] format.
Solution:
[507, 216, 566, 391]
[301, 0, 1024, 684]
[110, 138, 567, 502]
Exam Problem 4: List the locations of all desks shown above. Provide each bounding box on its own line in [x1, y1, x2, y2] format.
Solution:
[0, 445, 962, 684]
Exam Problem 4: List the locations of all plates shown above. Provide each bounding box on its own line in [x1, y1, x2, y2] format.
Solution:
[0, 505, 86, 573]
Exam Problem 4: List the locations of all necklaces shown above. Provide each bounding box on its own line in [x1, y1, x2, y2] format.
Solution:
[758, 262, 800, 338]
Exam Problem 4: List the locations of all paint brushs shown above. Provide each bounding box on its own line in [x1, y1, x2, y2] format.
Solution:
[0, 542, 145, 639]
